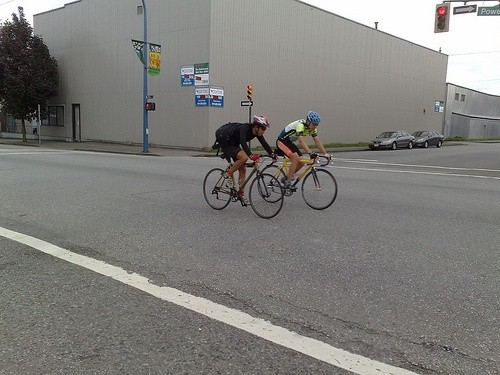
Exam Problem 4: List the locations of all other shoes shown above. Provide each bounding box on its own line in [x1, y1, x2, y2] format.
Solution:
[237, 195, 248, 203]
[295, 185, 298, 189]
[284, 182, 298, 189]
[224, 174, 234, 189]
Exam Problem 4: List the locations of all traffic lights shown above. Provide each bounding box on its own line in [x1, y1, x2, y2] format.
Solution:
[146, 103, 155, 111]
[246, 85, 252, 98]
[434, 4, 450, 32]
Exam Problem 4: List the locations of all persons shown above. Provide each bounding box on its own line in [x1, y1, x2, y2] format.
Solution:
[215, 115, 277, 204]
[276, 110, 330, 190]
[31, 117, 38, 139]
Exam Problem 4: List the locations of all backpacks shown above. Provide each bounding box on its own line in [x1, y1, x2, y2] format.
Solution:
[216, 122, 241, 146]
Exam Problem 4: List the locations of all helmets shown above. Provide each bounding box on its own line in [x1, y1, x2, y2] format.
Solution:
[253, 114, 270, 126]
[306, 111, 320, 124]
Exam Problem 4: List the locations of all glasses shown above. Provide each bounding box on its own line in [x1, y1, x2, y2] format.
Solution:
[259, 126, 266, 129]
[312, 123, 319, 126]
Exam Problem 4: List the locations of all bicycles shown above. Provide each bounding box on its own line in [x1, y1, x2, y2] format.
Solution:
[202, 154, 284, 219]
[257, 152, 338, 210]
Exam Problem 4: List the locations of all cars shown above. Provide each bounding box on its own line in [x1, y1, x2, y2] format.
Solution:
[411, 130, 444, 148]
[368, 130, 417, 150]
